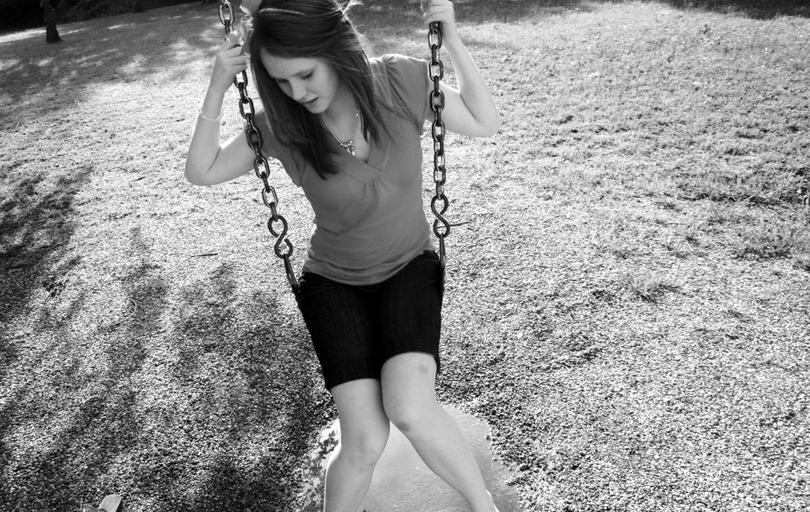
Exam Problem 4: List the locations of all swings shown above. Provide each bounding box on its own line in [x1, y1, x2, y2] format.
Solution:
[217, 0, 446, 325]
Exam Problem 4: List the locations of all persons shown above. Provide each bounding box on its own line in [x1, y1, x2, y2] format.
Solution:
[184, 0, 500, 512]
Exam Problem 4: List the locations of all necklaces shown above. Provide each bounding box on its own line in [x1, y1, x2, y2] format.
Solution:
[319, 108, 359, 156]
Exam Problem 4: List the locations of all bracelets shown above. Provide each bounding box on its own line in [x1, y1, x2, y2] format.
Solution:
[197, 107, 223, 123]
